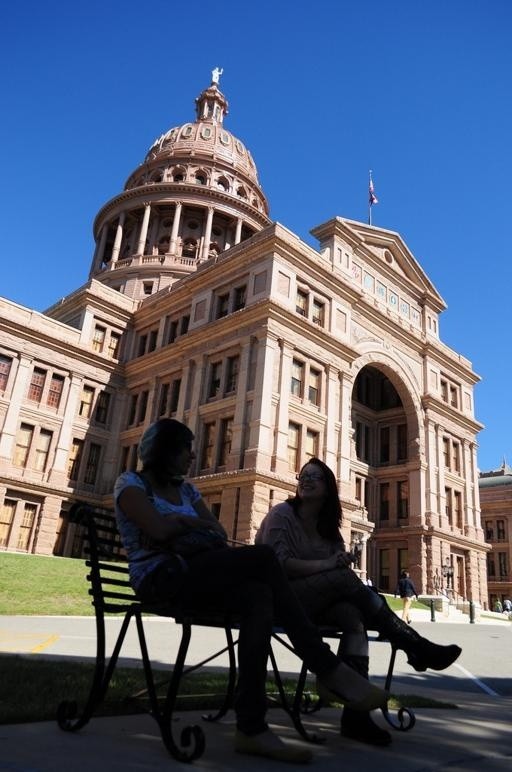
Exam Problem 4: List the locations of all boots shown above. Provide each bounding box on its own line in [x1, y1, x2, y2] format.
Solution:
[340, 654, 393, 746]
[366, 600, 462, 672]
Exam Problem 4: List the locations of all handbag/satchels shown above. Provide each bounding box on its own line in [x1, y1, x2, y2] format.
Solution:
[174, 524, 226, 546]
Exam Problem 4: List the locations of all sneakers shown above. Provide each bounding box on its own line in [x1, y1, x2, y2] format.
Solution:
[317, 663, 390, 713]
[232, 729, 314, 763]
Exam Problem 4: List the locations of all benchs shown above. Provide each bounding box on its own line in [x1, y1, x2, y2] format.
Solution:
[54, 499, 416, 762]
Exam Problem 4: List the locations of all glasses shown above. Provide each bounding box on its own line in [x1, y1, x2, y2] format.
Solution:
[296, 474, 324, 482]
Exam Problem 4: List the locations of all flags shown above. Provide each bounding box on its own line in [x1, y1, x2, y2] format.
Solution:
[368, 173, 379, 208]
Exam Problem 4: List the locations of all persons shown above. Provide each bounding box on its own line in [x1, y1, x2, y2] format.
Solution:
[398, 569, 419, 625]
[494, 598, 504, 613]
[503, 595, 512, 612]
[366, 576, 372, 586]
[252, 456, 463, 749]
[113, 418, 396, 765]
[210, 66, 225, 84]
